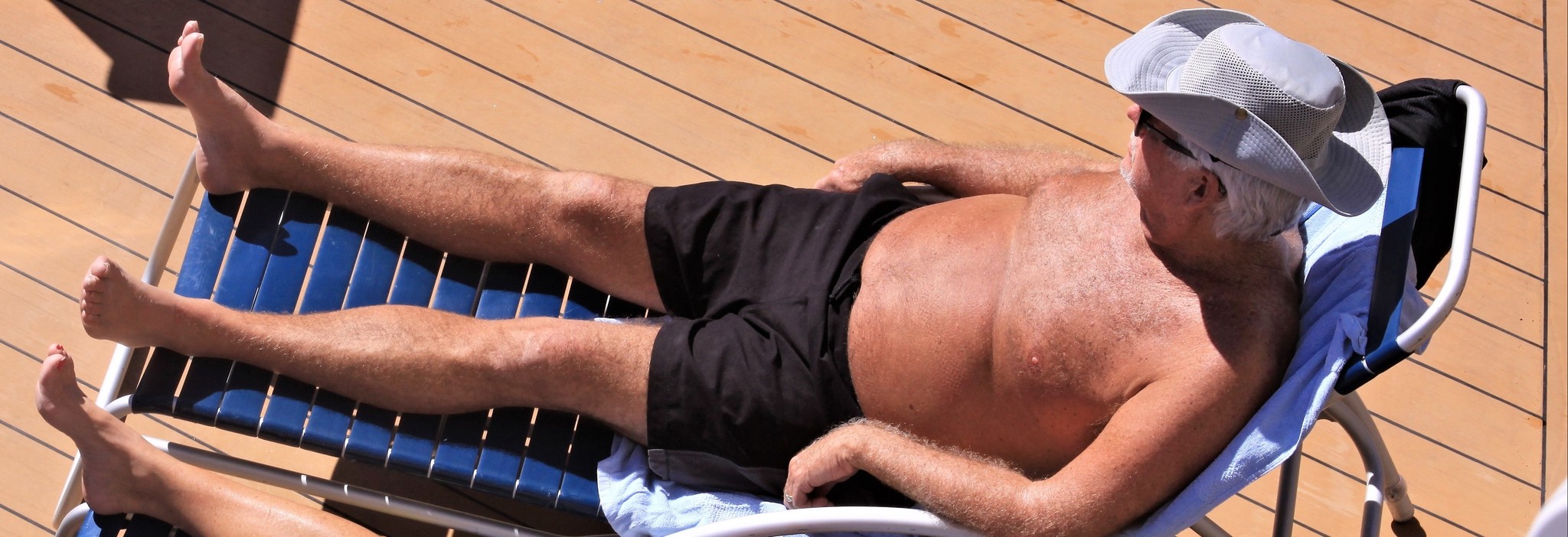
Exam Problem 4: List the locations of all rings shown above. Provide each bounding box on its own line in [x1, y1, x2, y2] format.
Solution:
[783, 490, 793, 503]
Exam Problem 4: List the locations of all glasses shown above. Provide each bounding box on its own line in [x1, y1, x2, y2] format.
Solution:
[1132, 108, 1227, 197]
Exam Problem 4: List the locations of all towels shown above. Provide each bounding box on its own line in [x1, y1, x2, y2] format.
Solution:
[593, 87, 1384, 537]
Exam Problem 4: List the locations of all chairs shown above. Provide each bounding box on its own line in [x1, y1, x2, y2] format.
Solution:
[49, 74, 1486, 536]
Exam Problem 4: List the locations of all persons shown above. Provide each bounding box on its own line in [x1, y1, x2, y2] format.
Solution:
[70, 8, 1390, 536]
[33, 347, 387, 537]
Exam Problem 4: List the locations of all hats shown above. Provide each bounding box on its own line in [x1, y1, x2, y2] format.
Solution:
[1104, 7, 1393, 219]
